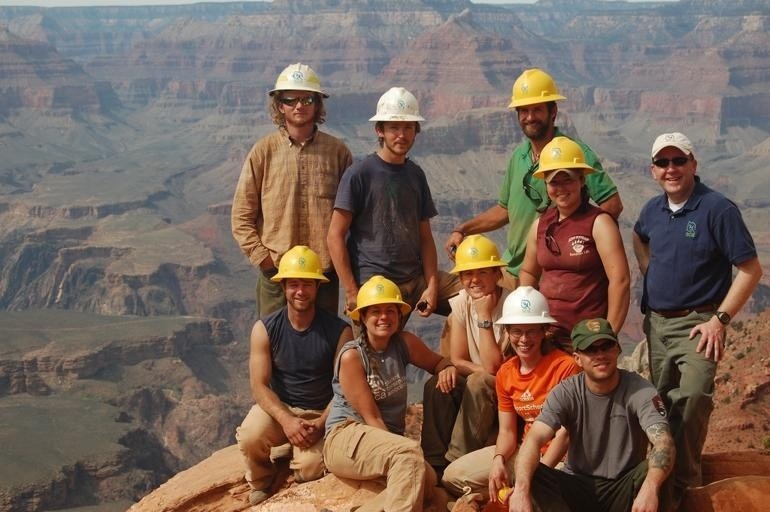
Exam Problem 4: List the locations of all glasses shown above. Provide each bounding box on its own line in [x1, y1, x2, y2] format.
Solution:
[280, 95, 316, 107]
[653, 157, 689, 167]
[522, 167, 543, 202]
[579, 341, 616, 353]
[544, 222, 561, 255]
[509, 327, 541, 338]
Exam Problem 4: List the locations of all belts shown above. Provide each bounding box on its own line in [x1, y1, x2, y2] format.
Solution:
[658, 304, 720, 318]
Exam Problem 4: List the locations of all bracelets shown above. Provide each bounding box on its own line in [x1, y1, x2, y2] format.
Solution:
[440, 364, 457, 372]
[494, 454, 506, 465]
[450, 227, 466, 237]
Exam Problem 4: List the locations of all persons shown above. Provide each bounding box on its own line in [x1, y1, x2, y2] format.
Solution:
[507, 317, 678, 512]
[418, 234, 514, 488]
[234, 244, 356, 506]
[518, 136, 632, 357]
[319, 273, 461, 512]
[443, 68, 625, 291]
[231, 61, 351, 323]
[440, 285, 585, 511]
[631, 131, 763, 512]
[325, 85, 466, 361]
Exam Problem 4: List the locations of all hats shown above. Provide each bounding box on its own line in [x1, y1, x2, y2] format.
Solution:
[651, 132, 697, 160]
[544, 168, 580, 183]
[570, 317, 618, 352]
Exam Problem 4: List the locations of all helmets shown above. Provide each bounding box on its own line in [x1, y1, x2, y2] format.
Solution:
[449, 233, 508, 275]
[532, 136, 594, 179]
[368, 87, 426, 122]
[507, 69, 568, 108]
[348, 275, 411, 320]
[267, 62, 330, 98]
[269, 245, 331, 284]
[495, 285, 558, 325]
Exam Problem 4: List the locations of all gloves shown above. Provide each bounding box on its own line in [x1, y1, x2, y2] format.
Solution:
[447, 486, 483, 512]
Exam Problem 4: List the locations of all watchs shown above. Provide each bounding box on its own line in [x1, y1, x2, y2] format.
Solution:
[714, 311, 731, 325]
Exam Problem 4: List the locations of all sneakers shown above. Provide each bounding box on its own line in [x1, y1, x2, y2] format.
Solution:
[248, 488, 272, 505]
